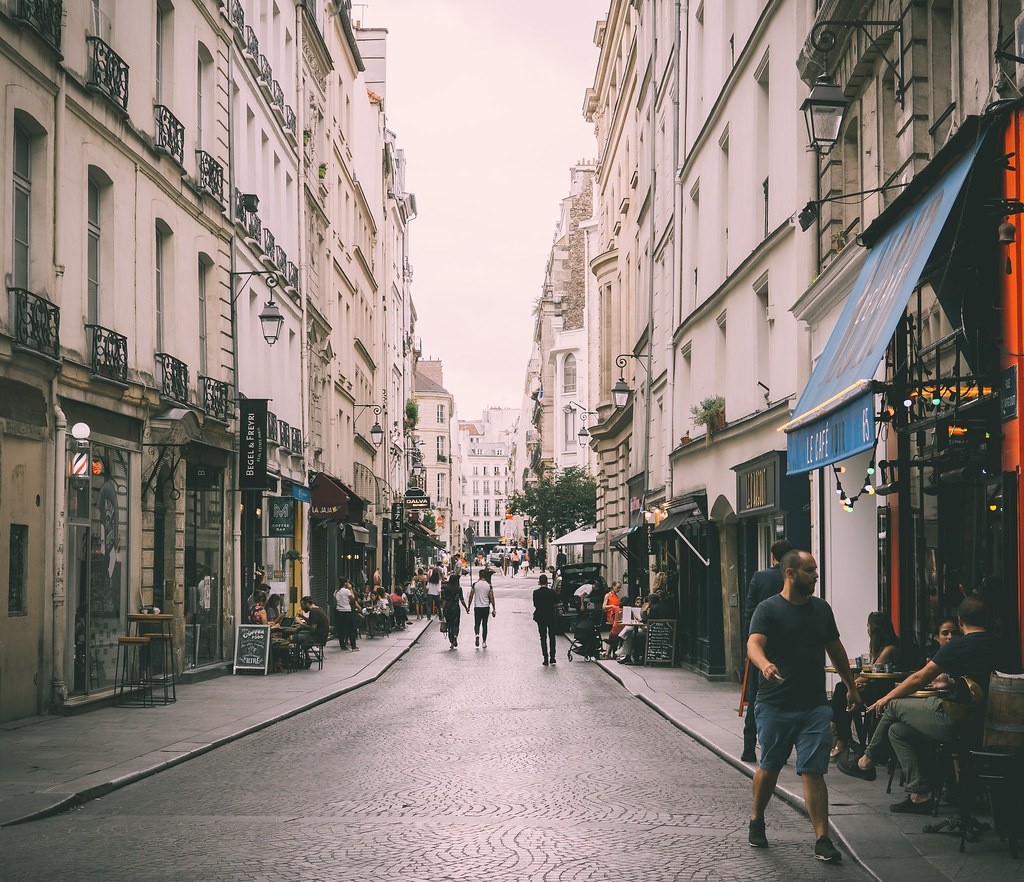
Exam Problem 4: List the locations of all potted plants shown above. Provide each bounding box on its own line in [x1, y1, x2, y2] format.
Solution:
[319, 162, 328, 178]
[304, 128, 312, 146]
[688, 393, 726, 433]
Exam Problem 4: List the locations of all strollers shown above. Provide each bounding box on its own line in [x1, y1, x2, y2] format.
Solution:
[567, 607, 606, 661]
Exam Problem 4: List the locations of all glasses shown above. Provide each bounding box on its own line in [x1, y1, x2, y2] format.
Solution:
[942, 630, 961, 636]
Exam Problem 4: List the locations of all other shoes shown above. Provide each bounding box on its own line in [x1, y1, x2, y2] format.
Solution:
[427, 618, 431, 620]
[550, 659, 556, 663]
[407, 621, 413, 624]
[439, 617, 444, 621]
[476, 637, 479, 646]
[829, 746, 846, 762]
[742, 752, 757, 762]
[483, 644, 487, 648]
[618, 658, 632, 664]
[421, 616, 424, 618]
[352, 647, 359, 652]
[341, 648, 350, 652]
[543, 662, 548, 665]
[305, 659, 312, 668]
[417, 617, 419, 619]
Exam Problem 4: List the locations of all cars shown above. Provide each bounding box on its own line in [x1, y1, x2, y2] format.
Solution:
[550, 562, 611, 636]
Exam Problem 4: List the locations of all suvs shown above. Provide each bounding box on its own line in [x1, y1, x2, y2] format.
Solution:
[489, 545, 529, 567]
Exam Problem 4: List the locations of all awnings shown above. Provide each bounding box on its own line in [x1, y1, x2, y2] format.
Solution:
[403, 521, 446, 549]
[609, 532, 639, 561]
[311, 471, 363, 521]
[777, 123, 998, 475]
[651, 510, 709, 566]
[550, 525, 598, 545]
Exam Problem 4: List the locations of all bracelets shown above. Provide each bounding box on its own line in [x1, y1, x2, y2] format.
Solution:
[468, 607, 470, 608]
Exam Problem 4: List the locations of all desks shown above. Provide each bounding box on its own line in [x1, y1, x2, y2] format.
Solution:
[119, 612, 177, 708]
[861, 666, 908, 771]
[826, 661, 862, 751]
[362, 608, 390, 637]
[606, 621, 623, 659]
[618, 623, 649, 666]
[276, 622, 302, 669]
[889, 676, 957, 811]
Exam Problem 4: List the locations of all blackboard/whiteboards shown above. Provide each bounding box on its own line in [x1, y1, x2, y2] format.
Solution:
[645, 617, 677, 662]
[234, 623, 270, 668]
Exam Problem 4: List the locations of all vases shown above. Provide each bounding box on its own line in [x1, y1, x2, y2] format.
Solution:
[681, 436, 689, 444]
[242, 194, 261, 212]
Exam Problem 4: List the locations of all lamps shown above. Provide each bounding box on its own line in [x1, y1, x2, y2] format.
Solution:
[563, 400, 586, 414]
[351, 402, 386, 448]
[72, 420, 94, 448]
[68, 447, 92, 478]
[576, 411, 598, 449]
[406, 451, 424, 478]
[786, 17, 907, 151]
[230, 271, 285, 348]
[789, 184, 908, 232]
[610, 351, 654, 414]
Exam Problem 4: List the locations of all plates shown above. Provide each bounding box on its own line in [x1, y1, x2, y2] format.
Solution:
[876, 670, 895, 673]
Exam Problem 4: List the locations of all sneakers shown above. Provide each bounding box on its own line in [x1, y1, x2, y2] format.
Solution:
[749, 819, 768, 846]
[814, 838, 842, 861]
[890, 794, 931, 815]
[836, 758, 876, 781]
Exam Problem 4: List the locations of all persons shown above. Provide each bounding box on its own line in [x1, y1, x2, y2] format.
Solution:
[246, 583, 329, 670]
[442, 574, 468, 648]
[468, 570, 496, 648]
[746, 549, 861, 861]
[533, 549, 567, 665]
[474, 544, 546, 583]
[741, 540, 794, 761]
[602, 571, 673, 666]
[830, 611, 901, 759]
[925, 617, 957, 684]
[837, 596, 1008, 814]
[573, 582, 601, 622]
[334, 554, 462, 652]
[374, 568, 380, 586]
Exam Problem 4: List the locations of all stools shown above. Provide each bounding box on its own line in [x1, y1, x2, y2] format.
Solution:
[302, 642, 326, 671]
[139, 631, 176, 703]
[274, 641, 296, 675]
[112, 634, 155, 711]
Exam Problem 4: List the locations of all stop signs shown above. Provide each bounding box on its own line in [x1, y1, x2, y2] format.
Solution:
[505, 509, 513, 520]
[437, 516, 443, 527]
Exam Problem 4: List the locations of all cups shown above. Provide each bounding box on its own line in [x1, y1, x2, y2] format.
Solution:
[885, 664, 895, 673]
[855, 657, 862, 667]
[861, 654, 872, 673]
[142, 610, 148, 614]
[874, 663, 883, 670]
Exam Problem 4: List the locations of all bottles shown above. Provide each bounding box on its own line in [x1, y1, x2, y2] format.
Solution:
[926, 657, 930, 664]
